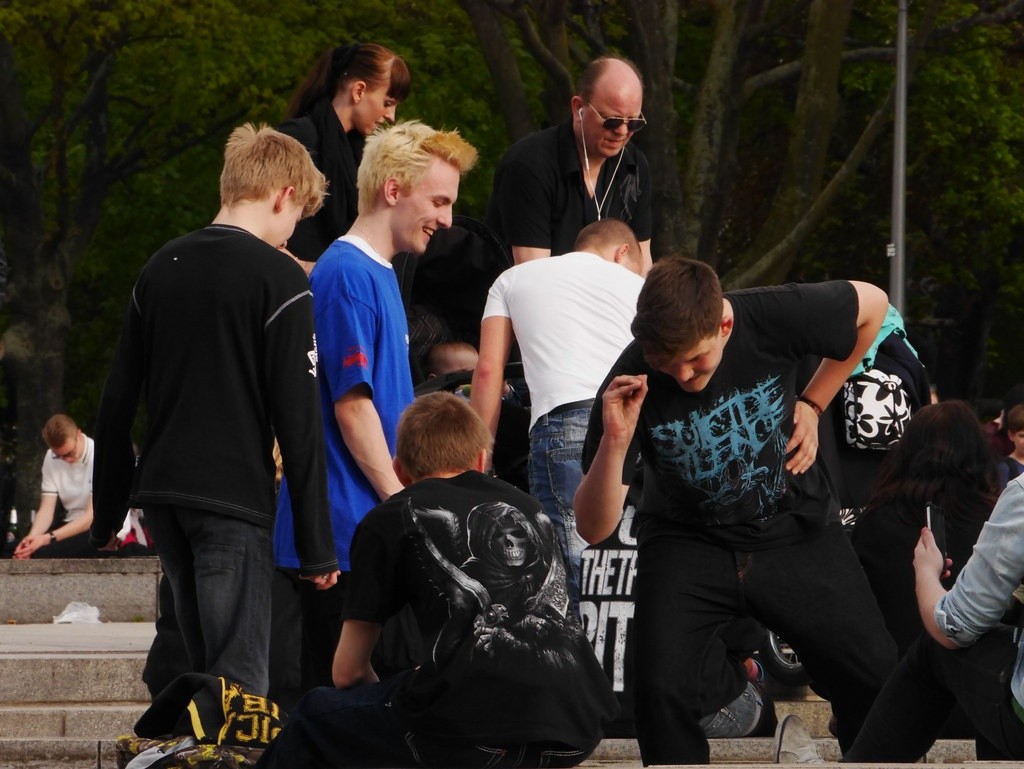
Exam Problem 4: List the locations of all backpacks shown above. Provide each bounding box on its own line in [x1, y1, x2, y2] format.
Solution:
[831, 368, 912, 462]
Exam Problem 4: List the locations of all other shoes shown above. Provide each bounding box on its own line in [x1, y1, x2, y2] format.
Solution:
[773, 714, 826, 764]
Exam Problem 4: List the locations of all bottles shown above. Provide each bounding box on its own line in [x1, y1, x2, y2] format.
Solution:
[7, 506, 19, 557]
[26, 508, 36, 534]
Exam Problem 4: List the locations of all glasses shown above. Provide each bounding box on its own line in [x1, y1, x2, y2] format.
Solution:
[579, 95, 647, 132]
[51, 430, 78, 459]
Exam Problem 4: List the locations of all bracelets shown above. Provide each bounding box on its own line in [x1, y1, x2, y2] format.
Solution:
[49, 531, 56, 543]
[797, 395, 824, 417]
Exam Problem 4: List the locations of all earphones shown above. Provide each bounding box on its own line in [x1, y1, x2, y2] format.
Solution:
[579, 107, 583, 120]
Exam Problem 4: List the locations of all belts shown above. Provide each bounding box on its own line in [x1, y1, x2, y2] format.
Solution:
[1010, 696, 1024, 722]
[536, 398, 595, 422]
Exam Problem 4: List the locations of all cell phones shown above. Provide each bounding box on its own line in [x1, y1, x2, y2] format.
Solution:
[926, 501, 948, 575]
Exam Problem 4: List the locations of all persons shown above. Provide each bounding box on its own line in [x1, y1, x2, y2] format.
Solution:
[572, 255, 908, 769]
[265, 118, 479, 715]
[816, 299, 930, 537]
[268, 42, 410, 493]
[472, 56, 780, 741]
[775, 392, 1024, 763]
[88, 121, 342, 702]
[426, 341, 517, 405]
[13, 413, 156, 558]
[252, 391, 623, 769]
